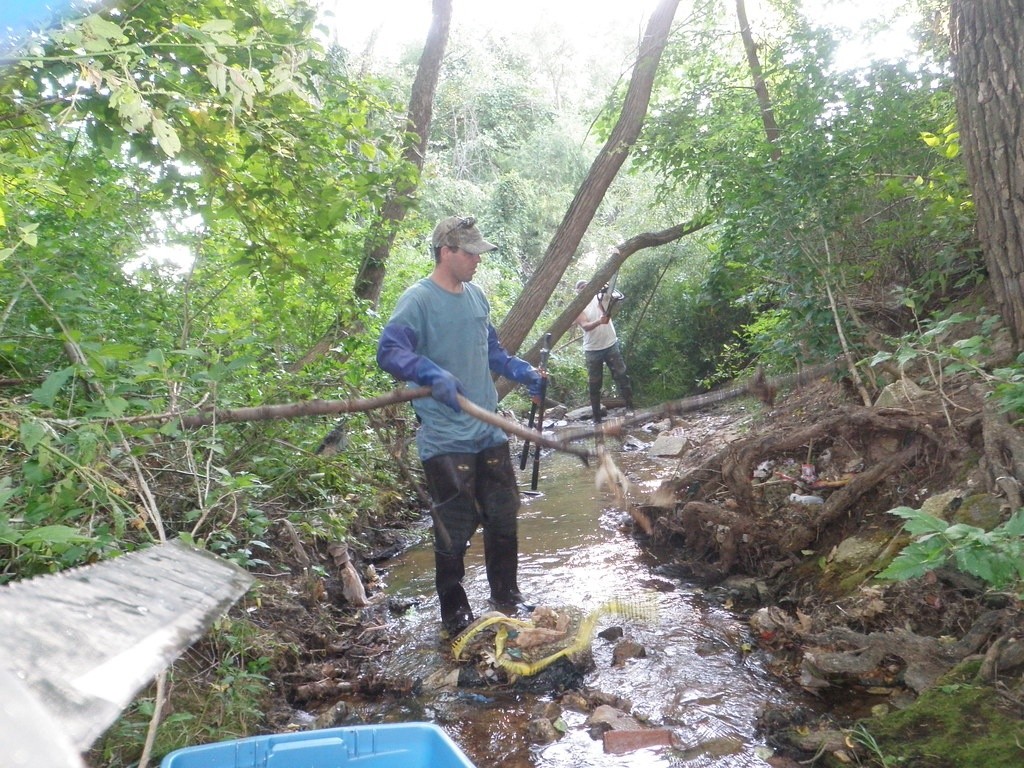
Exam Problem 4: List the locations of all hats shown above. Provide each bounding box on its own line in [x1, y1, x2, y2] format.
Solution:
[432, 216, 497, 254]
[575, 280, 588, 290]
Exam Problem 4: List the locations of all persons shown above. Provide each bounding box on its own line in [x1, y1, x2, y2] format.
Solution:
[574, 279, 635, 425]
[377, 215, 551, 643]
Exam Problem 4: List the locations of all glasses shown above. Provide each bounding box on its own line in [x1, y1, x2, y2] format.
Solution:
[446, 216, 478, 232]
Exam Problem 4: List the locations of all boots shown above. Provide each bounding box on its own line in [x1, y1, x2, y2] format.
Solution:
[622, 391, 635, 419]
[590, 393, 601, 424]
[481, 534, 533, 609]
[434, 553, 474, 635]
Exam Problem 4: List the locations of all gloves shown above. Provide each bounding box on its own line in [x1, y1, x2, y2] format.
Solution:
[376, 322, 467, 413]
[487, 321, 546, 397]
